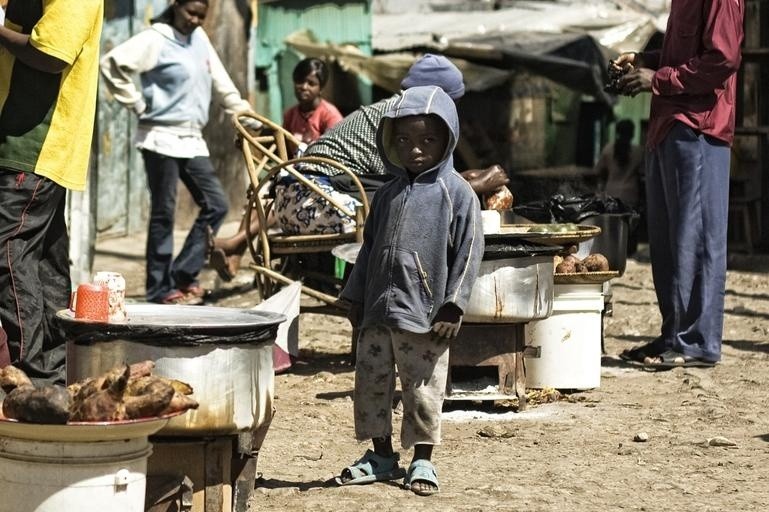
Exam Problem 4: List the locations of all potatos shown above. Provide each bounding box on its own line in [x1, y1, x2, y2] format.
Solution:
[554, 253, 609, 273]
[0, 360, 200, 423]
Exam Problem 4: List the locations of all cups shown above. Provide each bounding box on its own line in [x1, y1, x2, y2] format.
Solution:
[66, 283, 109, 322]
[90, 270, 127, 325]
[481, 208, 500, 234]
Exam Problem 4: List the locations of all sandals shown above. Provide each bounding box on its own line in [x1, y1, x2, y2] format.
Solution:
[205, 223, 237, 283]
[405, 458, 440, 496]
[160, 281, 206, 306]
[334, 448, 407, 487]
[618, 337, 717, 370]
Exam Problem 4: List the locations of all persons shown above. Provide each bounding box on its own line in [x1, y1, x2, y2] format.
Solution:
[594, 118, 646, 242]
[275, 52, 508, 234]
[282, 57, 346, 158]
[99, 0, 254, 304]
[1, 0, 106, 388]
[604, 1, 744, 369]
[334, 86, 486, 497]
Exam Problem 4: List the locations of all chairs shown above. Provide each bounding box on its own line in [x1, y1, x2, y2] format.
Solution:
[237, 107, 378, 313]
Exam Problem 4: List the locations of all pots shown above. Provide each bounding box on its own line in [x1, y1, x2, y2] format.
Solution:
[460, 251, 554, 322]
[0, 409, 192, 441]
[52, 302, 290, 435]
[573, 211, 640, 275]
